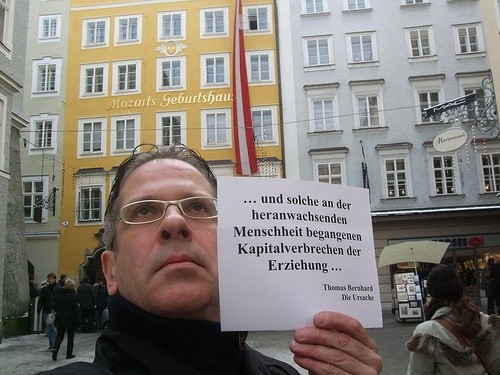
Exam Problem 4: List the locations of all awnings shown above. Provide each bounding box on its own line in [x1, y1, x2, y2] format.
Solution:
[371, 192, 500, 216]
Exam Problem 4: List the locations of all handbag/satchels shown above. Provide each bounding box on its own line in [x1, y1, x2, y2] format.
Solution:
[46, 314, 55, 324]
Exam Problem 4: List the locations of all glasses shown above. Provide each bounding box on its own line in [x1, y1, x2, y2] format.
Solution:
[112, 196, 217, 245]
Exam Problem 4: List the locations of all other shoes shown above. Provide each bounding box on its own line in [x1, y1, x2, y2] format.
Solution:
[48, 348, 54, 352]
[66, 354, 76, 359]
[52, 354, 57, 361]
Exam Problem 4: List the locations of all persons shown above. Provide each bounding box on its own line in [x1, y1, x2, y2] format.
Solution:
[76, 277, 107, 333]
[51, 279, 78, 361]
[406, 265, 500, 375]
[36, 142, 384, 375]
[30, 273, 67, 350]
[459, 258, 500, 316]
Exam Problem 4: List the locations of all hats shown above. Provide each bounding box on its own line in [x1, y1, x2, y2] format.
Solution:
[428, 265, 463, 303]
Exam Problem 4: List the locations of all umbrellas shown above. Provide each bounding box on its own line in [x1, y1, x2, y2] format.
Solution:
[378, 241, 451, 321]
